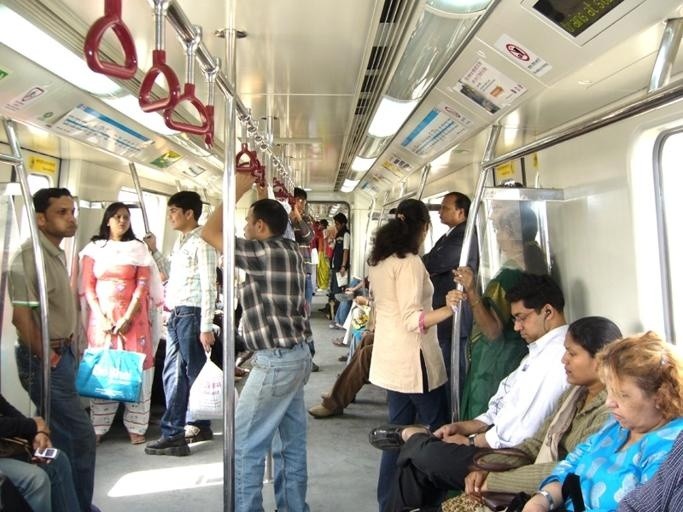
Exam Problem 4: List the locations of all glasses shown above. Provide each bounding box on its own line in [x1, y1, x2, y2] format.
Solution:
[512, 309, 536, 324]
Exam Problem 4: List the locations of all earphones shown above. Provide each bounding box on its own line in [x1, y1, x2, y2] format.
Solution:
[546, 309, 551, 316]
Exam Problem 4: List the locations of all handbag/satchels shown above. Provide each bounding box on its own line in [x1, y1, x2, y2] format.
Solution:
[466, 449, 584, 512]
[75, 335, 147, 402]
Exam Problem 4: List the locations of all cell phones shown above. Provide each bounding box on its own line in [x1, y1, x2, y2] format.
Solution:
[34, 448, 61, 461]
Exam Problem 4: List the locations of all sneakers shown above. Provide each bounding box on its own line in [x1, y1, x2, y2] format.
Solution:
[128, 426, 213, 457]
[370, 425, 431, 452]
[329, 321, 349, 361]
[309, 392, 355, 417]
[235, 367, 250, 382]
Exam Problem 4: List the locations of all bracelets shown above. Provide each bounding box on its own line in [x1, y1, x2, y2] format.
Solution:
[123, 316, 133, 325]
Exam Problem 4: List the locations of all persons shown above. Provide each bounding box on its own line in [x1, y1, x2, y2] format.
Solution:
[78, 199, 157, 446]
[0, 475, 40, 512]
[153, 167, 683, 510]
[0, 393, 81, 512]
[7, 188, 99, 512]
